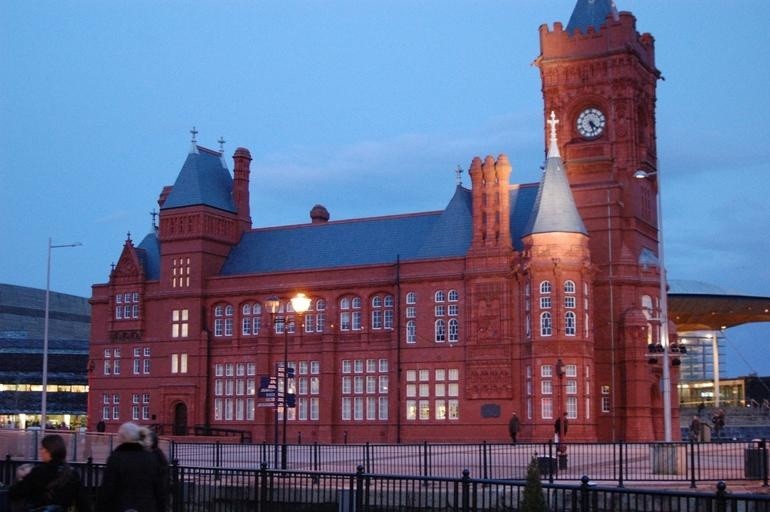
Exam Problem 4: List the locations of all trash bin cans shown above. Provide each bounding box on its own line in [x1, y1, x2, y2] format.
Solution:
[744, 439, 770, 480]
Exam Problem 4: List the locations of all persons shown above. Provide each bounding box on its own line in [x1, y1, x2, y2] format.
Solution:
[688, 416, 699, 442]
[508, 412, 520, 445]
[99, 423, 170, 512]
[0, 435, 81, 512]
[712, 411, 724, 437]
[554, 411, 568, 449]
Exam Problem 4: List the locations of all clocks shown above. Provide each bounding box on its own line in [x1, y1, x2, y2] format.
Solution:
[573, 106, 607, 141]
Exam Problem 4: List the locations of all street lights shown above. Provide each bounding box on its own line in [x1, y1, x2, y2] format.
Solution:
[633, 169, 686, 475]
[37, 237, 83, 447]
[555, 359, 569, 469]
[262, 291, 312, 476]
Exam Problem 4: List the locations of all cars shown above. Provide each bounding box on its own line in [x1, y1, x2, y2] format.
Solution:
[46, 419, 61, 429]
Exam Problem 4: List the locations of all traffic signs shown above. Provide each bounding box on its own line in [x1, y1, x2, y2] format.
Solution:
[256, 364, 297, 408]
